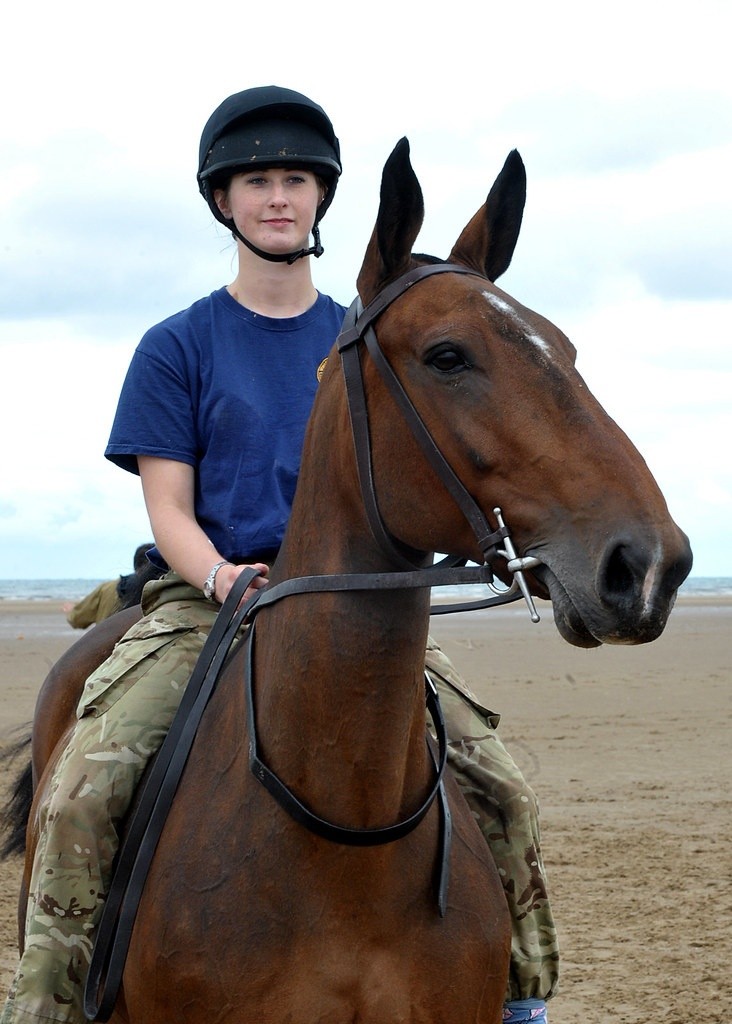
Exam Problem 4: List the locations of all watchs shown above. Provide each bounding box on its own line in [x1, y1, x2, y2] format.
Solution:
[202, 561, 237, 604]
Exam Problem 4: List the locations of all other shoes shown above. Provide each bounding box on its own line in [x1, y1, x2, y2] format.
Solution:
[502, 1000, 548, 1024]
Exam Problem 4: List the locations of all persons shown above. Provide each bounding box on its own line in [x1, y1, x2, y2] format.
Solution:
[3, 85, 561, 1024]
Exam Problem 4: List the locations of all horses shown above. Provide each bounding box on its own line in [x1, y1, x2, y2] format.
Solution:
[1, 138, 700, 1024]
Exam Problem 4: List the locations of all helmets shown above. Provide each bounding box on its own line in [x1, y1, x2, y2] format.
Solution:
[197, 87, 343, 224]
